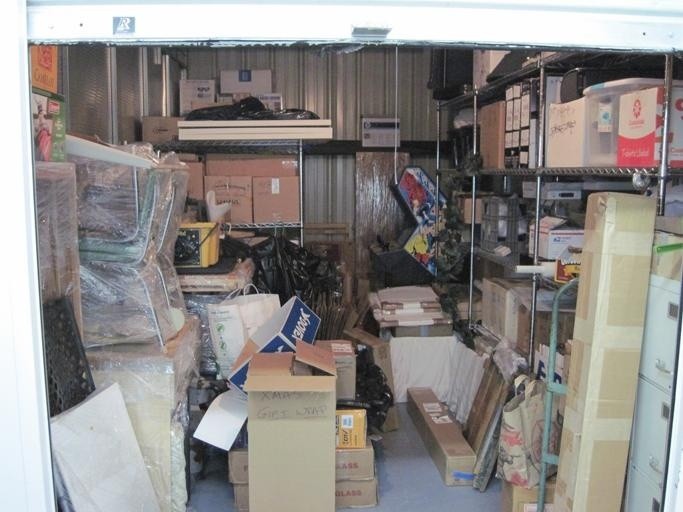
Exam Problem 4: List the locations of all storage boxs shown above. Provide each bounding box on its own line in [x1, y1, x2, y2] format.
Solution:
[30, 46, 682, 510]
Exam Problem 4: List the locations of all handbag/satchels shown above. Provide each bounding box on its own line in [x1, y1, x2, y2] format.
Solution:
[495, 375, 566, 490]
[206, 283, 281, 380]
